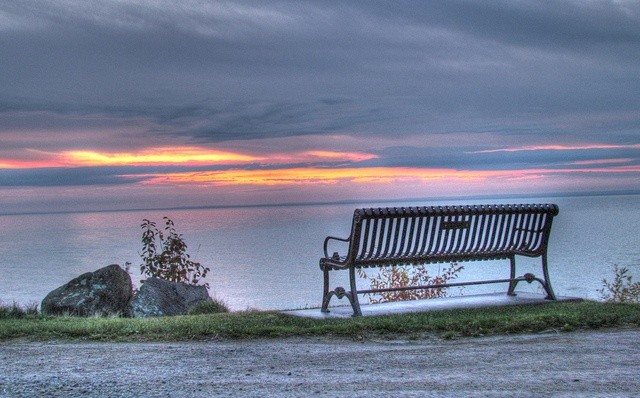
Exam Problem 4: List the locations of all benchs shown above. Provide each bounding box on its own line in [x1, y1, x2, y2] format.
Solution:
[319, 204, 559, 306]
